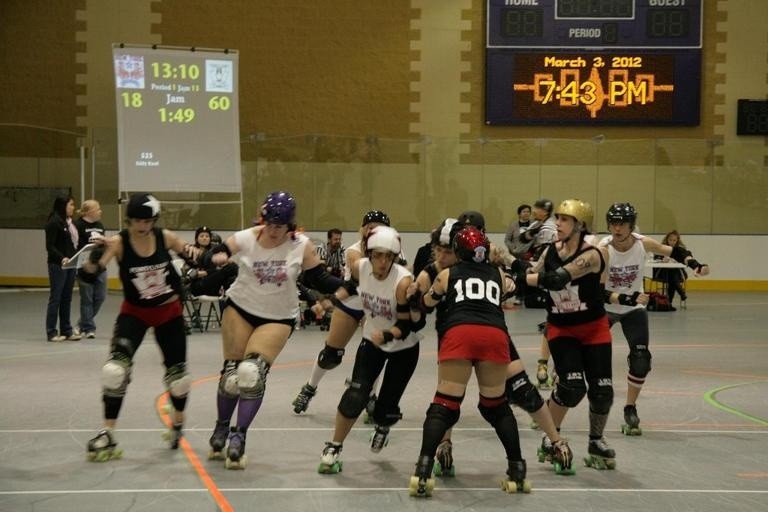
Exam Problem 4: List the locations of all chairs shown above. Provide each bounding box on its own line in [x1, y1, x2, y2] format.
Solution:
[170, 259, 222, 333]
[652, 254, 686, 311]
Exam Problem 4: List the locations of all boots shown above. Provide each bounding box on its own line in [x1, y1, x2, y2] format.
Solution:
[675, 283, 687, 300]
[668, 287, 677, 311]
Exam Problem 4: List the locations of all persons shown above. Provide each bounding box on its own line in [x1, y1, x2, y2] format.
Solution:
[82, 194, 229, 453]
[73, 199, 106, 339]
[44, 194, 83, 343]
[177, 191, 709, 496]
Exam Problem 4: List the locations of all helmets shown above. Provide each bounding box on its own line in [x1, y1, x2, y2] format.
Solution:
[456, 225, 490, 264]
[362, 210, 390, 226]
[606, 202, 636, 222]
[457, 210, 486, 235]
[126, 192, 161, 218]
[433, 217, 464, 248]
[534, 199, 553, 212]
[366, 226, 401, 254]
[260, 190, 296, 225]
[555, 197, 593, 233]
[196, 226, 212, 239]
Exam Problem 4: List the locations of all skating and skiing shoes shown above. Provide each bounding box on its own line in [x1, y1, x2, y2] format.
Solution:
[369, 424, 390, 453]
[225, 426, 248, 470]
[318, 440, 343, 473]
[433, 440, 454, 477]
[162, 404, 184, 449]
[619, 405, 641, 435]
[364, 394, 380, 424]
[552, 440, 576, 475]
[292, 382, 318, 415]
[409, 456, 435, 497]
[500, 459, 531, 493]
[583, 433, 616, 469]
[208, 420, 229, 460]
[537, 359, 551, 390]
[88, 428, 124, 463]
[191, 312, 203, 330]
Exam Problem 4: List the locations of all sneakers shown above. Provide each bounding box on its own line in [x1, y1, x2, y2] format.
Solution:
[87, 330, 96, 338]
[60, 332, 82, 340]
[48, 336, 62, 342]
[72, 321, 81, 336]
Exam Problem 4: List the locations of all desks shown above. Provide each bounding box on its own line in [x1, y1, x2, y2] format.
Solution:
[647, 258, 686, 269]
